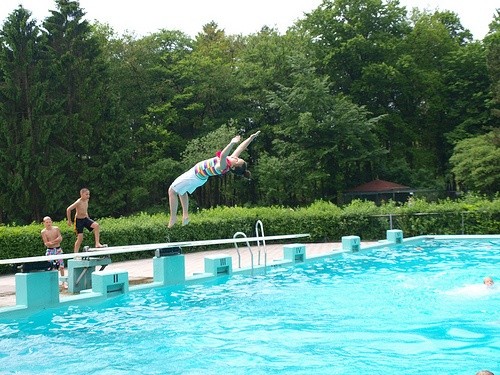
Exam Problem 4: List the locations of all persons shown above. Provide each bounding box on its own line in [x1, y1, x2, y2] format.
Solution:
[67, 189, 103, 260]
[476, 370, 494, 375]
[484, 278, 494, 285]
[41, 217, 64, 289]
[168, 130, 261, 227]
